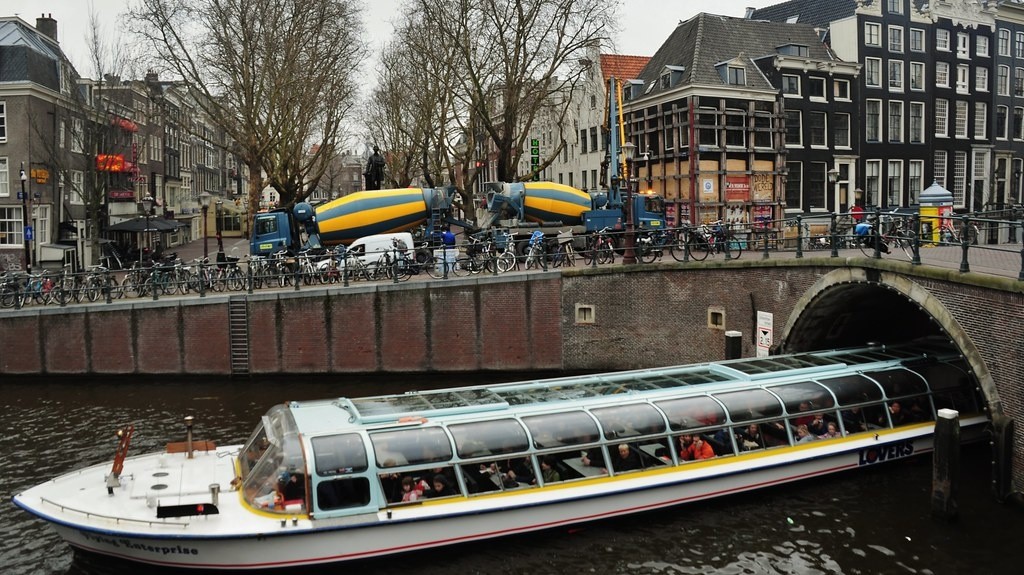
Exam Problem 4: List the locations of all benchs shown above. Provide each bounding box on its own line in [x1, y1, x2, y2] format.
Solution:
[390, 385, 928, 501]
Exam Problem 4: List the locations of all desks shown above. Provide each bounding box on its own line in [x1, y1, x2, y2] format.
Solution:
[669, 415, 707, 428]
[828, 412, 883, 430]
[639, 441, 685, 467]
[617, 425, 642, 437]
[488, 470, 530, 490]
[562, 457, 605, 477]
[534, 436, 565, 446]
[709, 433, 760, 450]
[752, 410, 798, 432]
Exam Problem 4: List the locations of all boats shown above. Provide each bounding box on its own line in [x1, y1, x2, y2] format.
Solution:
[11, 337, 991, 575]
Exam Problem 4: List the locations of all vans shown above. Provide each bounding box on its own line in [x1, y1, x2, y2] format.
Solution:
[332, 231, 416, 279]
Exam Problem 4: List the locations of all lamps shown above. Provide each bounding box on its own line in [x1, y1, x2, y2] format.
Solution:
[992, 167, 1000, 180]
[1012, 167, 1024, 179]
[643, 152, 651, 161]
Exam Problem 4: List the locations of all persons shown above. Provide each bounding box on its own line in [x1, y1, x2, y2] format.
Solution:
[365, 146, 385, 190]
[797, 401, 815, 424]
[441, 227, 455, 249]
[844, 408, 867, 433]
[482, 197, 487, 209]
[155, 241, 164, 254]
[853, 204, 863, 222]
[820, 422, 849, 439]
[124, 241, 138, 258]
[808, 414, 827, 436]
[883, 404, 900, 427]
[248, 401, 784, 502]
[795, 425, 815, 444]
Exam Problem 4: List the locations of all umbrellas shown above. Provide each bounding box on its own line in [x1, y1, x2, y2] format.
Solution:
[105, 216, 178, 265]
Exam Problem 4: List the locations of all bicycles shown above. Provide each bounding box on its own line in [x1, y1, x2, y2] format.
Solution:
[669, 221, 711, 261]
[857, 207, 916, 261]
[0, 225, 616, 314]
[687, 217, 742, 261]
[930, 211, 980, 247]
[636, 225, 690, 264]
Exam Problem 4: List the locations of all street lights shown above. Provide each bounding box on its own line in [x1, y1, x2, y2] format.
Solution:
[827, 167, 840, 257]
[198, 191, 212, 289]
[141, 191, 154, 259]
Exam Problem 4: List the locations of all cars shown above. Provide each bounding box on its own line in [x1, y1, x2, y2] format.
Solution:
[304, 246, 347, 275]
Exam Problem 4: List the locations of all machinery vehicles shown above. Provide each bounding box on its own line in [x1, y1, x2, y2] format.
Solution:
[249, 186, 478, 263]
[472, 181, 667, 264]
